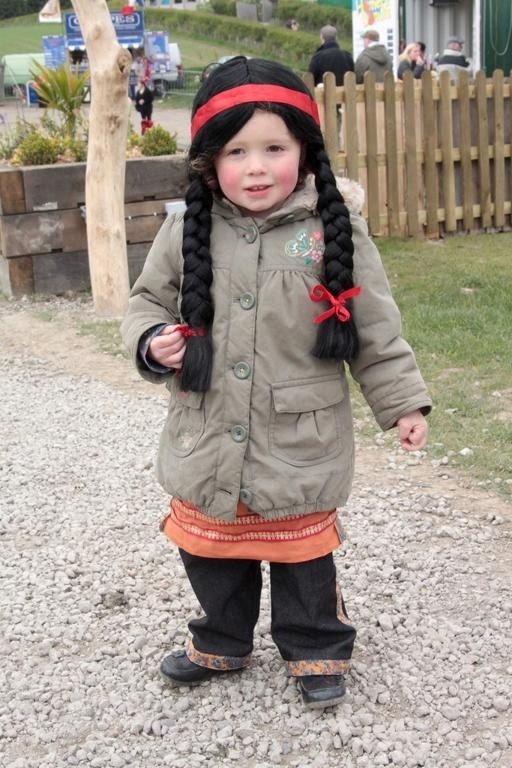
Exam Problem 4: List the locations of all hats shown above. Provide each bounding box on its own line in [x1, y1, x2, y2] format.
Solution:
[445, 36, 466, 45]
[361, 30, 379, 42]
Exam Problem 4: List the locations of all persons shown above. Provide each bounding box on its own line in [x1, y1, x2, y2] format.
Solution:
[434, 36, 471, 81]
[307, 25, 356, 152]
[353, 29, 395, 84]
[134, 79, 154, 133]
[121, 58, 439, 705]
[397, 42, 426, 80]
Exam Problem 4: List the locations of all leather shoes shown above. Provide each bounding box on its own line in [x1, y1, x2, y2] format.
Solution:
[298, 675, 348, 708]
[160, 650, 230, 682]
